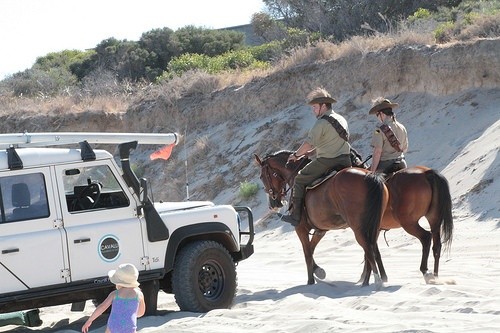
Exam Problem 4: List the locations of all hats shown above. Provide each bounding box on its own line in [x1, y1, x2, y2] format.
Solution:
[107, 263, 140, 288]
[305, 88, 337, 106]
[368, 98, 400, 115]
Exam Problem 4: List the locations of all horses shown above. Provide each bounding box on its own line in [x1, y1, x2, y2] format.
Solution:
[253, 148, 454, 287]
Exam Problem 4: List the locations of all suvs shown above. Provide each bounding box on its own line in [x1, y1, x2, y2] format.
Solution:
[0, 130, 258, 315]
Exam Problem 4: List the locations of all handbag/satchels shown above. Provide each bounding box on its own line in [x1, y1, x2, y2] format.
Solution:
[351, 148, 372, 169]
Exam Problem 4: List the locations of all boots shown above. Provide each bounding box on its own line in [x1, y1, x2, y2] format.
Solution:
[276, 197, 304, 226]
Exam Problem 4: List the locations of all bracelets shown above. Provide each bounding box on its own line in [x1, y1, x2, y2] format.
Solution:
[294, 153, 300, 158]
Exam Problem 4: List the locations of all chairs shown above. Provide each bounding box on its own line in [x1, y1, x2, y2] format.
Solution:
[11, 183, 34, 217]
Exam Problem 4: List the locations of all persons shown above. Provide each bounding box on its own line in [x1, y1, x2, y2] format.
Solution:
[278, 89, 352, 227]
[366, 97, 409, 176]
[82, 263, 145, 333]
